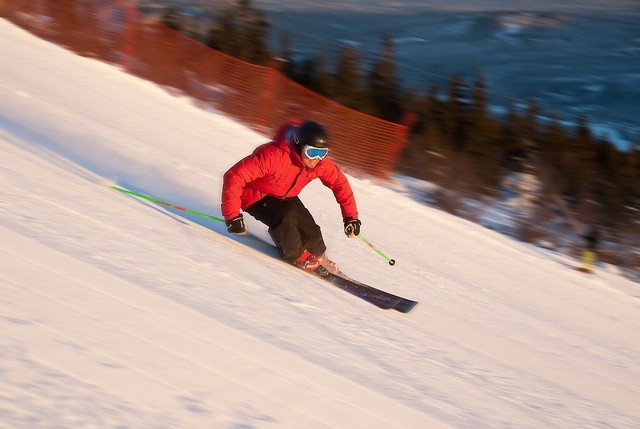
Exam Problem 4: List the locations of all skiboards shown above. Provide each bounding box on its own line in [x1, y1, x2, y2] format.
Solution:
[317, 267, 419, 314]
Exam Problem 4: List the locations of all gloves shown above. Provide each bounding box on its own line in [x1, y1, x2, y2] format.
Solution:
[224, 213, 245, 233]
[344, 219, 361, 238]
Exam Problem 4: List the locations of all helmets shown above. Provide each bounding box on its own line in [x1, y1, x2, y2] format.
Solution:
[293, 120, 329, 157]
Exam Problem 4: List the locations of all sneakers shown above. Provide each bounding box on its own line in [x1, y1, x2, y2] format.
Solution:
[293, 249, 320, 272]
[320, 256, 339, 276]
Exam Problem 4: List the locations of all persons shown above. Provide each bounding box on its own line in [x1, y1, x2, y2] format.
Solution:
[576, 221, 601, 273]
[220, 119, 362, 278]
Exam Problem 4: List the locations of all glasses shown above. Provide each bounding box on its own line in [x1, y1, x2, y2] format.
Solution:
[293, 133, 329, 160]
